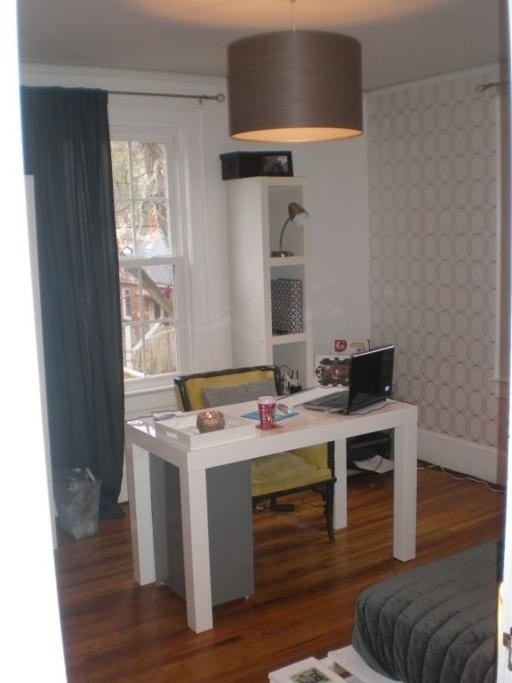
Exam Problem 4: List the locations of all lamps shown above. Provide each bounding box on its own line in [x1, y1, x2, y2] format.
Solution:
[173, 366, 337, 546]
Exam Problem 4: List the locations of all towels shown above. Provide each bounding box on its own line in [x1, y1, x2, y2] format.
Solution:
[54, 470, 101, 540]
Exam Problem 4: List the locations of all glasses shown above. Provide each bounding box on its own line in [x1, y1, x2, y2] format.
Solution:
[271, 202, 310, 257]
[225, 1, 363, 143]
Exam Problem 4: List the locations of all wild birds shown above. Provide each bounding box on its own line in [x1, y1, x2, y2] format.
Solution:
[303, 343, 395, 415]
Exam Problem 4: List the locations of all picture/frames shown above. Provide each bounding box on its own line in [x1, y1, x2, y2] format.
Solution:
[125, 386, 419, 637]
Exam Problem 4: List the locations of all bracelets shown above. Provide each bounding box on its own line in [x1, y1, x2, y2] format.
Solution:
[197, 411, 225, 433]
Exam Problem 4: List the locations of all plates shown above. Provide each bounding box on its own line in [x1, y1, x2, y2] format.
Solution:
[229, 177, 314, 397]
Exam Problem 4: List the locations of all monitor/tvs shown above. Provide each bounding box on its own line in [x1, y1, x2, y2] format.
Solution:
[268, 656, 347, 683]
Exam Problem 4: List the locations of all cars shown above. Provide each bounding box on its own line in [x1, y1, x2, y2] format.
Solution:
[258, 396, 276, 429]
